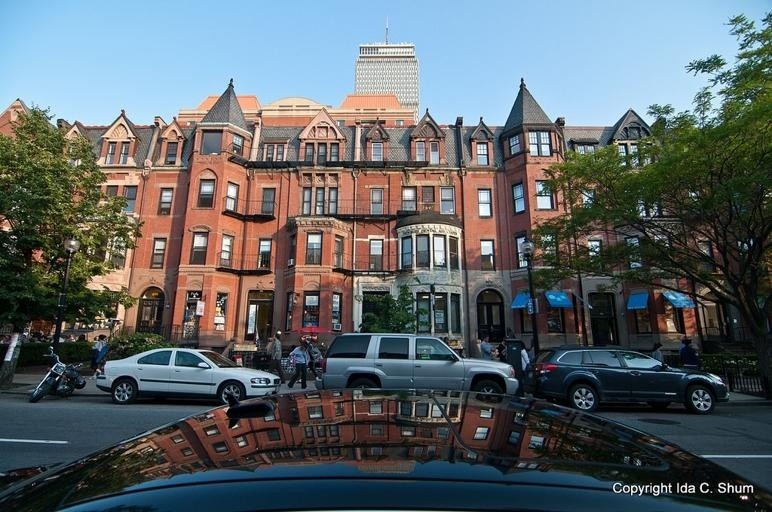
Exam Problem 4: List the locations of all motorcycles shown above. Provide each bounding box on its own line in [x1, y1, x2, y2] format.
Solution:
[29, 345, 86, 401]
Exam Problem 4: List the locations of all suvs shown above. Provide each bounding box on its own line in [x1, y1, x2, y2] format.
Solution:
[522, 344, 730, 416]
[315, 332, 520, 393]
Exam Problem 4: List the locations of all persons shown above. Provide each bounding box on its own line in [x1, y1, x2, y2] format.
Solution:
[480, 335, 495, 360]
[306, 340, 324, 381]
[89, 334, 107, 380]
[0, 323, 99, 344]
[678, 336, 704, 371]
[287, 341, 310, 391]
[519, 341, 530, 372]
[268, 332, 286, 384]
[653, 342, 665, 364]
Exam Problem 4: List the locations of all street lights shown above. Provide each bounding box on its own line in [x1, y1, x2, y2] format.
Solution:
[54, 236, 82, 354]
[521, 236, 539, 356]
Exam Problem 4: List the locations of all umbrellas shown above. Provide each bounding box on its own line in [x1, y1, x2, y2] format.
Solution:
[293, 327, 333, 335]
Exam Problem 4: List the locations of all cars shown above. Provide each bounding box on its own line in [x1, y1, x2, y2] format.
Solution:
[97, 347, 280, 404]
